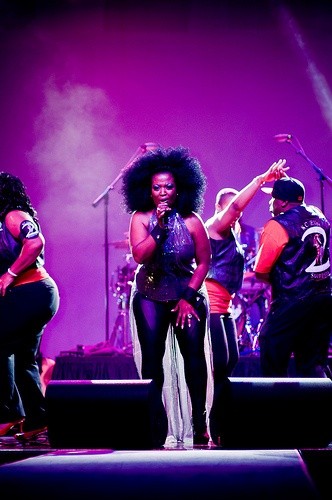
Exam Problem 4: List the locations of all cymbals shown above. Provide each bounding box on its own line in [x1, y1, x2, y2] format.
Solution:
[105, 239, 130, 249]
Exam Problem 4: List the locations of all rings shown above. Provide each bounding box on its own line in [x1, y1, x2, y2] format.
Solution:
[187, 314, 192, 318]
[157, 208, 160, 210]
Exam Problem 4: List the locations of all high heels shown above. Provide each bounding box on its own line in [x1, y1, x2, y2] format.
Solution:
[191, 412, 210, 444]
[15, 425, 48, 440]
[0, 418, 25, 435]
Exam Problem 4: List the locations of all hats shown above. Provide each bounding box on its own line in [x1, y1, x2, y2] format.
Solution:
[260, 177, 305, 204]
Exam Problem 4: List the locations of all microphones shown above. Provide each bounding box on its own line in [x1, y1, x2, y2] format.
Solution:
[163, 203, 170, 232]
[274, 133, 294, 142]
[141, 142, 161, 151]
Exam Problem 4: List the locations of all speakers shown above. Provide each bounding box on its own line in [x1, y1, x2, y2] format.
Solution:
[209, 375, 332, 449]
[45, 378, 168, 450]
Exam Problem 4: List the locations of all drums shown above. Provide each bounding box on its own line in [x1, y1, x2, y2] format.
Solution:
[230, 293, 247, 338]
[112, 264, 138, 311]
[238, 222, 268, 295]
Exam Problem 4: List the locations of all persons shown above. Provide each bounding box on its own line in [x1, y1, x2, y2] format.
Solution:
[205, 158, 289, 395]
[0, 173, 60, 446]
[254, 170, 332, 377]
[122, 146, 212, 443]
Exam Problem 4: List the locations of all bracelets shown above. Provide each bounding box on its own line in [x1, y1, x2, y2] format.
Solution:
[150, 225, 167, 244]
[8, 268, 18, 276]
[181, 287, 205, 307]
